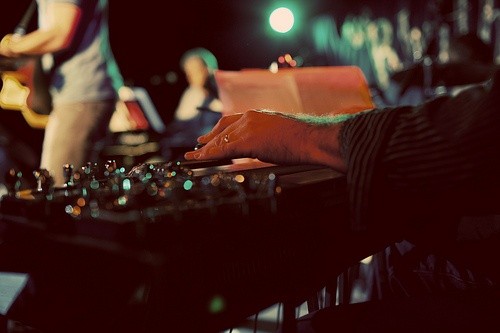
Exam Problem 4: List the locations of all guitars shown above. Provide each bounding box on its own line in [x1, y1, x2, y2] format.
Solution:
[0, 68, 49, 129]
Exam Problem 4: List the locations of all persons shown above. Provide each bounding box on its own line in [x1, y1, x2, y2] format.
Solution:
[174, 47, 220, 144]
[0, 0, 109, 187]
[177, 55, 500, 333]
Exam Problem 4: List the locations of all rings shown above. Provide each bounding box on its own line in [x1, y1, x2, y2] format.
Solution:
[224, 132, 230, 143]
